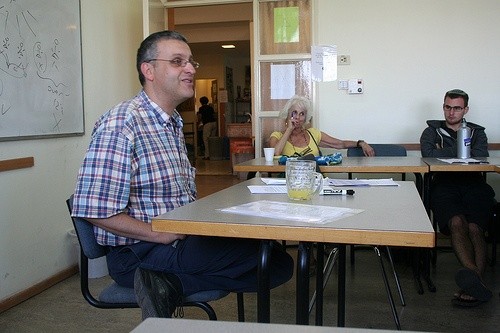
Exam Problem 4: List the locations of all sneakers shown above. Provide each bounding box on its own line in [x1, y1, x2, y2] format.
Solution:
[133, 267, 185, 322]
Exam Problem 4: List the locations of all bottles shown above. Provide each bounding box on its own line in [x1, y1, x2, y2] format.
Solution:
[457, 118, 471, 159]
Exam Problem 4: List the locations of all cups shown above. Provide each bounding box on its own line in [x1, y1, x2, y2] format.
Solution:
[263, 148, 275, 161]
[285, 160, 324, 201]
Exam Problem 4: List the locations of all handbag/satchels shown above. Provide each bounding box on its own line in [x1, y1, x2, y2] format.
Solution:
[278, 150, 344, 165]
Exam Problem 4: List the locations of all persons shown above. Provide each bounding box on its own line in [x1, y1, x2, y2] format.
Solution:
[196, 96, 218, 161]
[420, 89, 500, 307]
[268, 94, 375, 158]
[71, 31, 294, 321]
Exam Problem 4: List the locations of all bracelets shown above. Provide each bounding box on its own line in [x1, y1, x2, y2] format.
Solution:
[357, 140, 364, 148]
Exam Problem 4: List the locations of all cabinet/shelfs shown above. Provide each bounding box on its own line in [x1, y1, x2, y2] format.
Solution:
[234, 97, 251, 123]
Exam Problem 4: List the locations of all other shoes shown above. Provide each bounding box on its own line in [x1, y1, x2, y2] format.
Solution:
[309, 258, 316, 272]
[325, 247, 340, 259]
[454, 267, 494, 305]
[451, 289, 484, 308]
[202, 156, 209, 160]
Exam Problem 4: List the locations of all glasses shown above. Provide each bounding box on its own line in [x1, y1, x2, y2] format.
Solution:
[141, 55, 199, 69]
[443, 105, 465, 113]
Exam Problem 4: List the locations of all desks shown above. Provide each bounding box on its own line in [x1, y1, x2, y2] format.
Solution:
[232, 157, 429, 294]
[152, 177, 436, 329]
[421, 157, 500, 293]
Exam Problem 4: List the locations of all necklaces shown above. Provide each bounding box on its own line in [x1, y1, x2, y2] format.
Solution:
[290, 130, 313, 157]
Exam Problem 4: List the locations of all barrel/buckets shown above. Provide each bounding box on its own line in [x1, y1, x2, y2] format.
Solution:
[68, 229, 108, 278]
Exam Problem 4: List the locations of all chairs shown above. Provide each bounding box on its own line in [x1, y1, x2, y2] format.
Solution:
[347, 144, 407, 181]
[65, 193, 245, 322]
